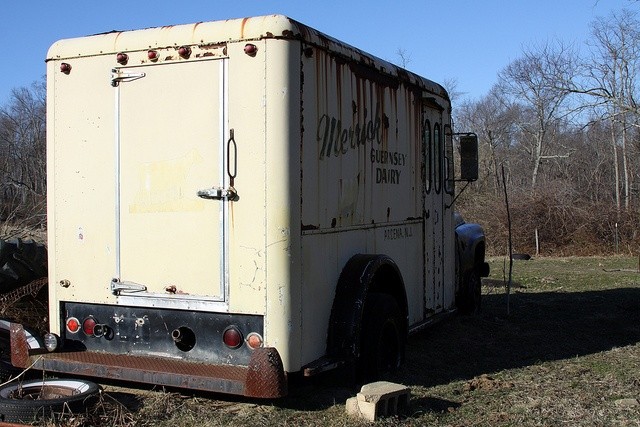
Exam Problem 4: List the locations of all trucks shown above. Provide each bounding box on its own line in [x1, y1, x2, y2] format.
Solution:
[10, 14, 486, 400]
